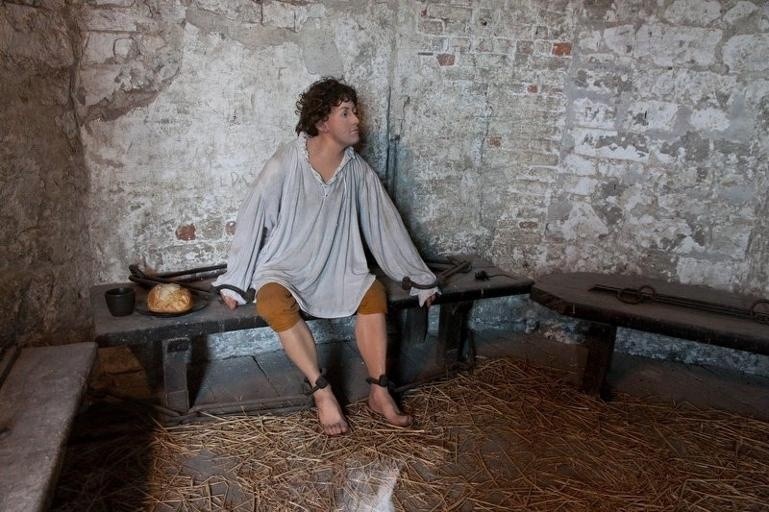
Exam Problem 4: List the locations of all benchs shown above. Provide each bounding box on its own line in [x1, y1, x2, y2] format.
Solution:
[532, 268, 765, 412]
[0, 339, 100, 511]
[93, 253, 532, 420]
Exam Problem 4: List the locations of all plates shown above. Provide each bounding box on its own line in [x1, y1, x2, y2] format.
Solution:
[134, 296, 207, 315]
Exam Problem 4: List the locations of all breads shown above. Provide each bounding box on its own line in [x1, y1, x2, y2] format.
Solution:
[146, 282, 193, 313]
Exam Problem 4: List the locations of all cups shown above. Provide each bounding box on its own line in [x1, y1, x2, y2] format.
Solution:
[104, 287, 135, 317]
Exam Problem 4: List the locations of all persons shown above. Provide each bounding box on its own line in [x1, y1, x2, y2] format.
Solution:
[212, 78, 442, 436]
